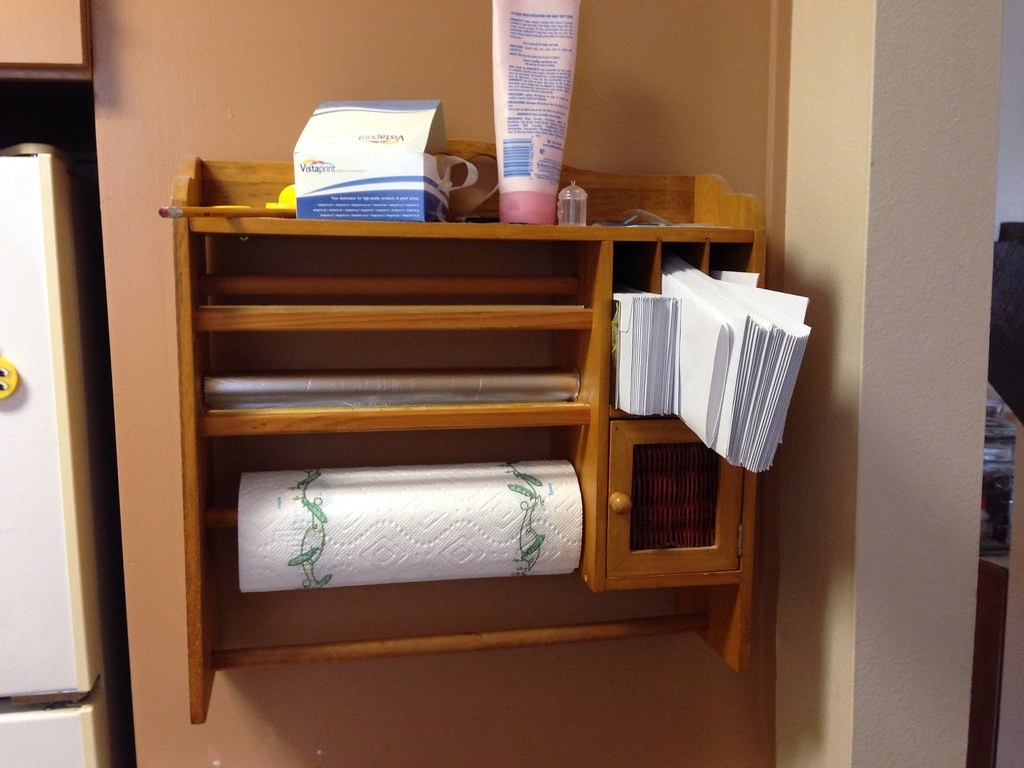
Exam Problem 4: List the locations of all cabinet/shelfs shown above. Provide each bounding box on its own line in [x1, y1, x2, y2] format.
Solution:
[157, 151, 766, 725]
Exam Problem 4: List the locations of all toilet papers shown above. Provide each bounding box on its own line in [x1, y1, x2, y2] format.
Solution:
[239, 461, 583, 593]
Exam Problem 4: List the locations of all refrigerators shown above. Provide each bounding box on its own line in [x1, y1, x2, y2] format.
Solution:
[0, 142, 138, 768]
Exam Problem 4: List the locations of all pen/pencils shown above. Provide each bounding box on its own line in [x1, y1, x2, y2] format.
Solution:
[158, 205, 297, 218]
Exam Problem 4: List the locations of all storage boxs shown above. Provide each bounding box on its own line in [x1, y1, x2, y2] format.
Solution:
[294, 101, 446, 221]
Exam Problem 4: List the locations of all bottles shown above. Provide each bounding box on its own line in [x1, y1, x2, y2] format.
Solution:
[557, 180, 588, 226]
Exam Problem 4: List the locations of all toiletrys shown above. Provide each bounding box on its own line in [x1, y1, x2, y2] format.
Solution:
[492, 0, 581, 225]
